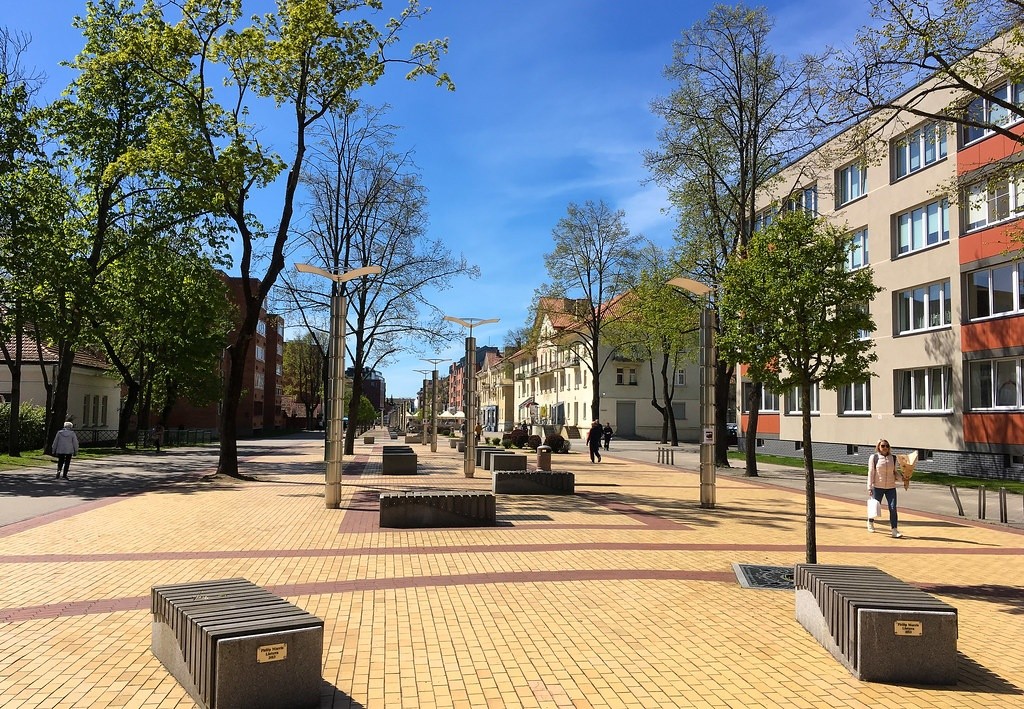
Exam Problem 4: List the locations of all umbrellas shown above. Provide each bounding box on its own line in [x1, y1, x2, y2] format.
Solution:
[342, 417, 348, 421]
[406, 410, 419, 423]
[437, 410, 465, 424]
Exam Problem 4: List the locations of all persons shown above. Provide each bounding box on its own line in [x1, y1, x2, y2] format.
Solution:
[587, 419, 613, 463]
[522, 420, 528, 432]
[867, 439, 909, 537]
[343, 421, 348, 432]
[475, 423, 482, 440]
[51, 422, 79, 480]
[460, 421, 466, 435]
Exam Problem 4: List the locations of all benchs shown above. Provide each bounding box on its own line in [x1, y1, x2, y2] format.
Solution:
[363, 436, 374, 444]
[383, 445, 418, 475]
[387, 427, 406, 439]
[492, 471, 575, 495]
[481, 450, 515, 470]
[379, 491, 496, 527]
[490, 453, 528, 474]
[475, 448, 505, 466]
[449, 438, 496, 452]
[150, 577, 324, 709]
[794, 562, 960, 684]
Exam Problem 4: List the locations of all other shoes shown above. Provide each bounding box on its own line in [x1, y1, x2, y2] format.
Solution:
[62, 477, 69, 480]
[891, 527, 903, 538]
[598, 456, 601, 463]
[56, 471, 60, 478]
[867, 520, 876, 533]
[591, 461, 594, 464]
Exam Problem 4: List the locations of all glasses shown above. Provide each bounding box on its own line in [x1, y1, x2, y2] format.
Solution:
[879, 444, 890, 449]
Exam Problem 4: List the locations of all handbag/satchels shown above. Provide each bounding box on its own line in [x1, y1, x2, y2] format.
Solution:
[867, 498, 882, 520]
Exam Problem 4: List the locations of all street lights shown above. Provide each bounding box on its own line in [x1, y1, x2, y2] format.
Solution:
[538, 344, 558, 424]
